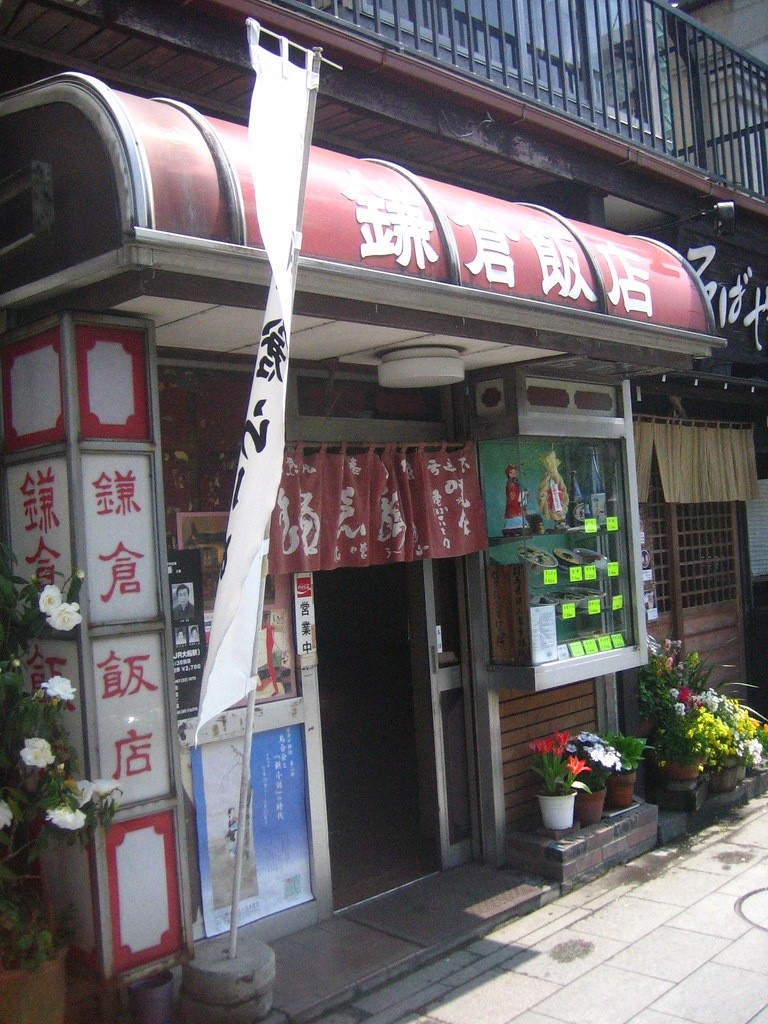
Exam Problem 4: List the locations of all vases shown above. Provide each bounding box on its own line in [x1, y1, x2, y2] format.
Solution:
[535, 790, 577, 831]
[660, 757, 707, 781]
[0, 945, 69, 1024]
[574, 785, 607, 827]
[709, 760, 739, 793]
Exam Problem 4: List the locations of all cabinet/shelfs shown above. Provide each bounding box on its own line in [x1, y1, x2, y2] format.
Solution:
[477, 434, 649, 693]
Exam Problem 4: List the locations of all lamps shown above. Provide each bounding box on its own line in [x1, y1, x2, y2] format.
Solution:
[378, 347, 465, 388]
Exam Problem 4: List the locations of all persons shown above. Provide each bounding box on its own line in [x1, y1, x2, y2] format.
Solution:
[172, 584, 194, 618]
[502, 464, 533, 529]
[175, 629, 187, 644]
[188, 627, 200, 643]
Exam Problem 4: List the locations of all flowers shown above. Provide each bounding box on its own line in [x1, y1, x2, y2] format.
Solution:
[527, 728, 593, 796]
[0, 542, 125, 976]
[634, 634, 716, 771]
[701, 686, 768, 772]
[565, 730, 622, 793]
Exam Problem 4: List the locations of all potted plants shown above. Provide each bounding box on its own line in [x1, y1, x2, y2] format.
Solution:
[595, 720, 655, 809]
